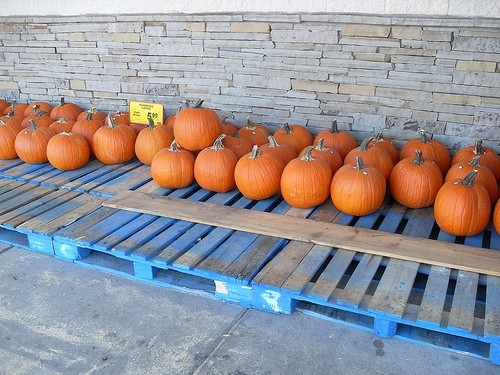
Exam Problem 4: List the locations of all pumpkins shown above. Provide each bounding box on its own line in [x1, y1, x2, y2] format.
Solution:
[0, 97, 500, 236]
